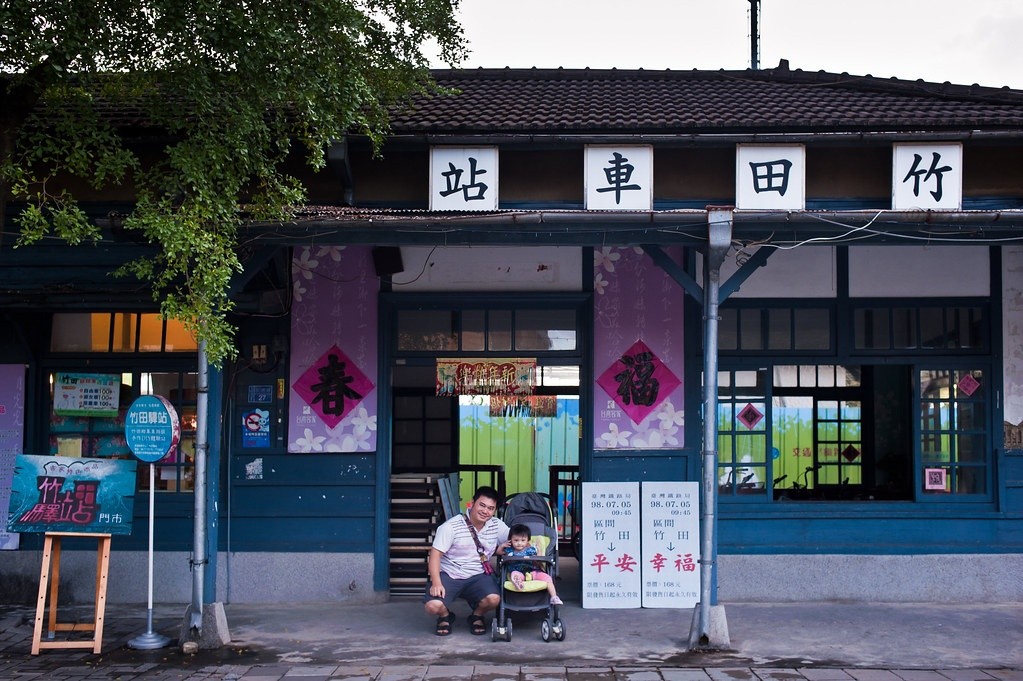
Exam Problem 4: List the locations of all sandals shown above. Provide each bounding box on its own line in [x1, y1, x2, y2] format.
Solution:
[467, 612, 486, 635]
[436, 607, 455, 636]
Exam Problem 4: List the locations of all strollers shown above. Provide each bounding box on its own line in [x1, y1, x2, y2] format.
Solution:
[490, 492, 566, 643]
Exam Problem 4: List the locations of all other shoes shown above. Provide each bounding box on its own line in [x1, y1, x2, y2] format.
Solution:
[550, 596, 563, 605]
[511, 570, 523, 590]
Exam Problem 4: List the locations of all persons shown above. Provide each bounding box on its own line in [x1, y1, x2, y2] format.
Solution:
[496, 524, 563, 605]
[423, 486, 542, 636]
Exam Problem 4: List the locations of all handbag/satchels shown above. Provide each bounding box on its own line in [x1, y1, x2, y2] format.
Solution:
[480, 553, 494, 575]
